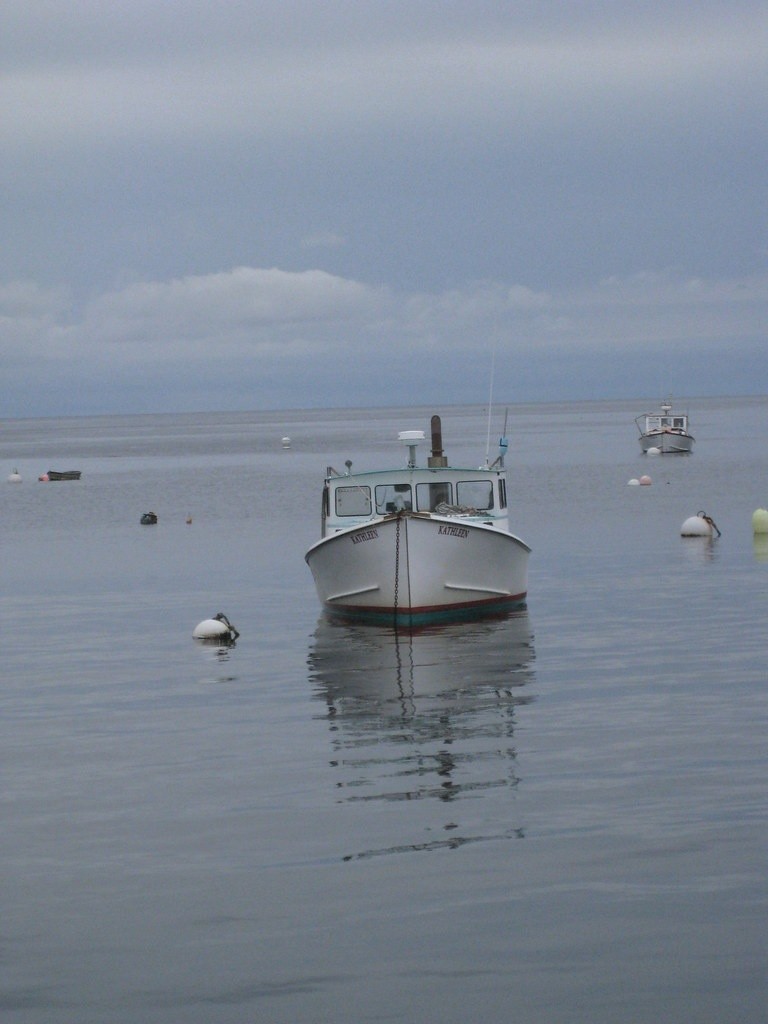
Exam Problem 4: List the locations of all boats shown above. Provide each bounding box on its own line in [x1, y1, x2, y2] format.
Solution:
[632, 389, 697, 455]
[303, 346, 535, 627]
